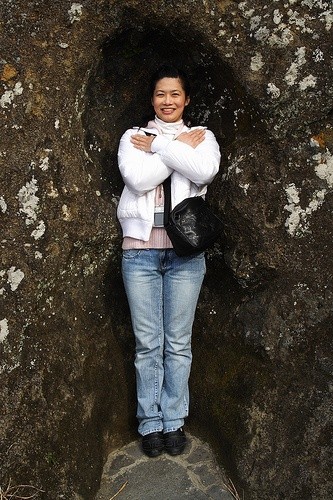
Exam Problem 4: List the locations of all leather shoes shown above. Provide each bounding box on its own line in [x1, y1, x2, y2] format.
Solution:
[163, 425, 187, 456]
[142, 431, 164, 457]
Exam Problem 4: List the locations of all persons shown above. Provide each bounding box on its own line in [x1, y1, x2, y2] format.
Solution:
[116, 65, 221, 458]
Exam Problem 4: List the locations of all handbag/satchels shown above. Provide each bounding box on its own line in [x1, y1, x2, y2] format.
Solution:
[164, 195, 227, 259]
